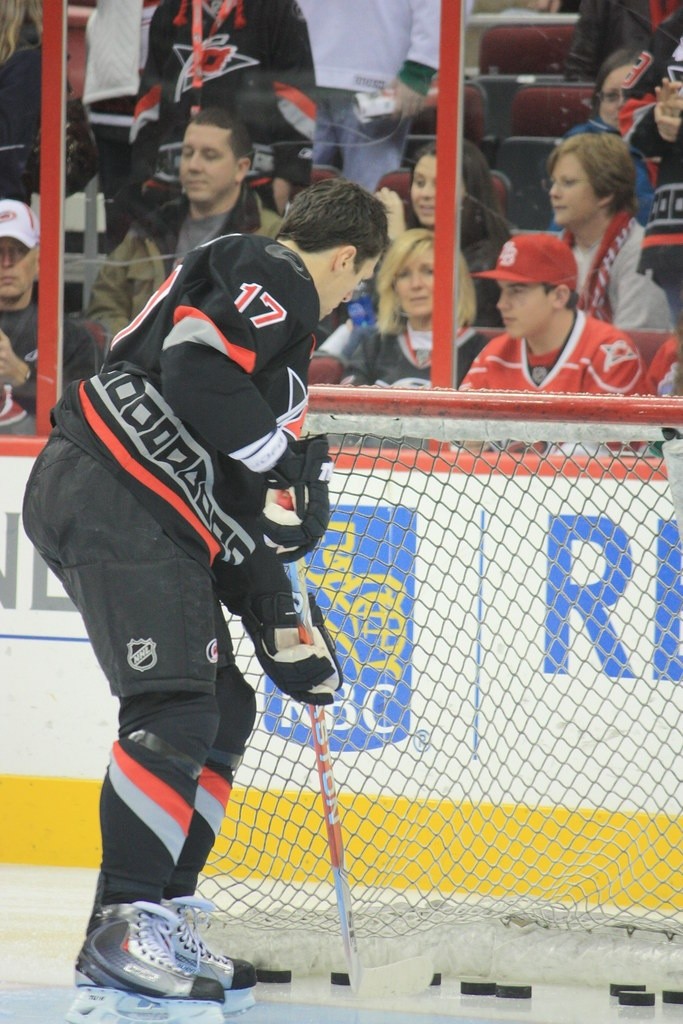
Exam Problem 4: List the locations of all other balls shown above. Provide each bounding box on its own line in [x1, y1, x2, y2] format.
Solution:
[430, 973, 442, 986]
[662, 988, 683, 1004]
[610, 983, 647, 996]
[495, 985, 532, 998]
[331, 971, 349, 985]
[460, 982, 497, 995]
[257, 968, 293, 984]
[619, 990, 655, 1006]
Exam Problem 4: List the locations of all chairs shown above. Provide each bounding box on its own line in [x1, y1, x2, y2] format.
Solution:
[374, 169, 515, 222]
[403, 82, 498, 163]
[498, 80, 596, 232]
[474, 11, 582, 118]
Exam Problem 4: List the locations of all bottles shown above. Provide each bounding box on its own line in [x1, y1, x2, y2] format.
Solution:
[347, 280, 377, 332]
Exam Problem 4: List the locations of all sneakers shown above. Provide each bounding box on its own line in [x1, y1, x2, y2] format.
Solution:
[160, 897, 256, 1019]
[65, 901, 225, 1024]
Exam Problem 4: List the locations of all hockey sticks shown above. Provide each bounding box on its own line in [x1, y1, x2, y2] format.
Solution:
[271, 486, 363, 996]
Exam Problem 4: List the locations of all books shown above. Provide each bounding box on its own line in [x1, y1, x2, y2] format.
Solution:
[351, 77, 440, 125]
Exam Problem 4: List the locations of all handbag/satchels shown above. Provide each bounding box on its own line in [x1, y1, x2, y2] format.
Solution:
[20, 96, 104, 199]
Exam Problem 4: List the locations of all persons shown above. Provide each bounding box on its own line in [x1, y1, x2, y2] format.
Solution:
[23, 179, 389, 1005]
[0, 0, 683, 457]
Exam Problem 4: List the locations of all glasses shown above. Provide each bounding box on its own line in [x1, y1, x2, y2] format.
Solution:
[595, 91, 624, 103]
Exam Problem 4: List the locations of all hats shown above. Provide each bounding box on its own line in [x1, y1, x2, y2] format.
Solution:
[469, 235, 577, 289]
[0, 199, 39, 248]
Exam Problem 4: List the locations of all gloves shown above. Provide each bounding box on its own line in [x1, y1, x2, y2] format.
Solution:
[240, 591, 343, 705]
[257, 433, 332, 564]
